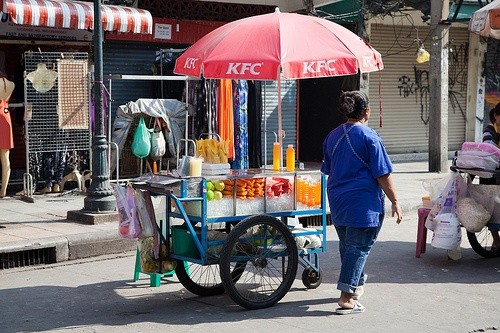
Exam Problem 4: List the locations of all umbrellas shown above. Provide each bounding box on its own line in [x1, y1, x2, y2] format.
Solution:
[172, 6, 384, 169]
[469, 0, 500, 39]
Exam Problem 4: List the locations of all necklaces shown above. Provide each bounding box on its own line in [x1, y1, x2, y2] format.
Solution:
[348, 117, 364, 124]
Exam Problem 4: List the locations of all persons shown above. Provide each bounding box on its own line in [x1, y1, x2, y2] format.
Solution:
[15, 63, 79, 195]
[0, 70, 15, 198]
[479, 101, 500, 252]
[320, 90, 403, 315]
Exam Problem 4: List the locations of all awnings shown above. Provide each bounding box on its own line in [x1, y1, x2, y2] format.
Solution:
[4, 0, 153, 33]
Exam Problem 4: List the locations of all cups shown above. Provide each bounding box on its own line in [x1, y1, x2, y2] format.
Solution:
[190, 157, 202, 176]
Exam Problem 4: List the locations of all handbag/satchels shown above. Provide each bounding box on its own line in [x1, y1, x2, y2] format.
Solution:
[150, 118, 166, 163]
[131, 116, 151, 160]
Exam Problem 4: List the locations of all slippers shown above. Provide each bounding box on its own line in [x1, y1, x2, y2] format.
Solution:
[336, 301, 366, 314]
[352, 285, 363, 300]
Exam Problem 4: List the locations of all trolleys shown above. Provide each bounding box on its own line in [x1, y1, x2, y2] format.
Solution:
[121, 170, 327, 310]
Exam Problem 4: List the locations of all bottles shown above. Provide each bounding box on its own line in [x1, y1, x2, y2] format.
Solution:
[286, 144, 295, 172]
[272, 142, 282, 173]
[297, 176, 322, 209]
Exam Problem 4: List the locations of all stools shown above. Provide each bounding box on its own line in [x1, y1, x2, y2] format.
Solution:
[133, 244, 190, 288]
[416, 207, 432, 258]
